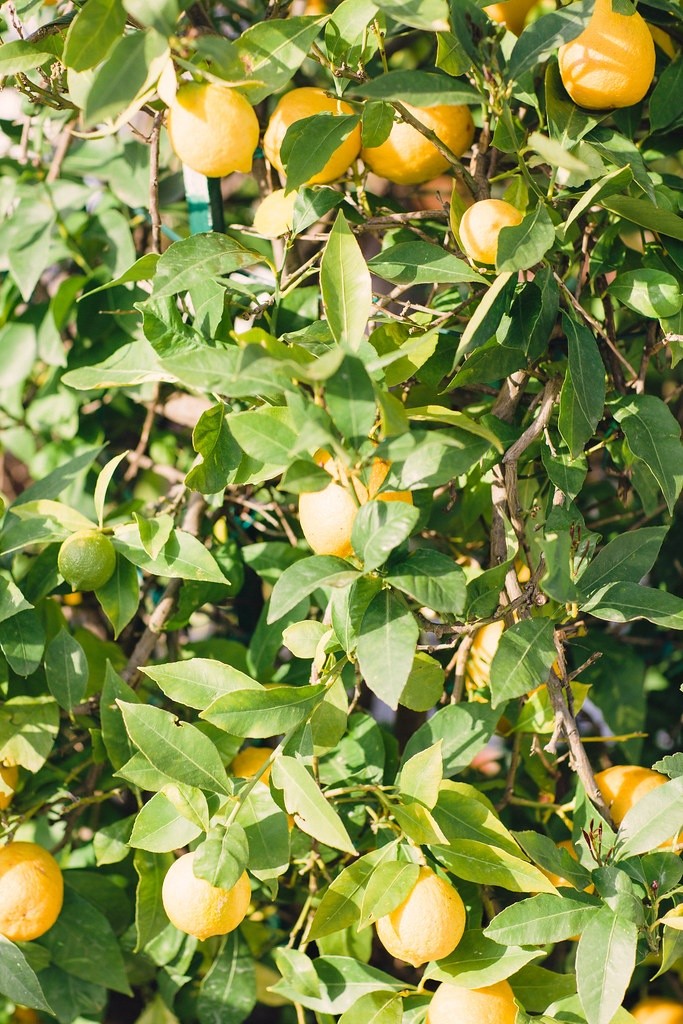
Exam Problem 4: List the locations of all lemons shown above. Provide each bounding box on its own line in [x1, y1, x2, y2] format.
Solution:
[163, 0, 655, 266]
[0, 457, 683, 1024]
